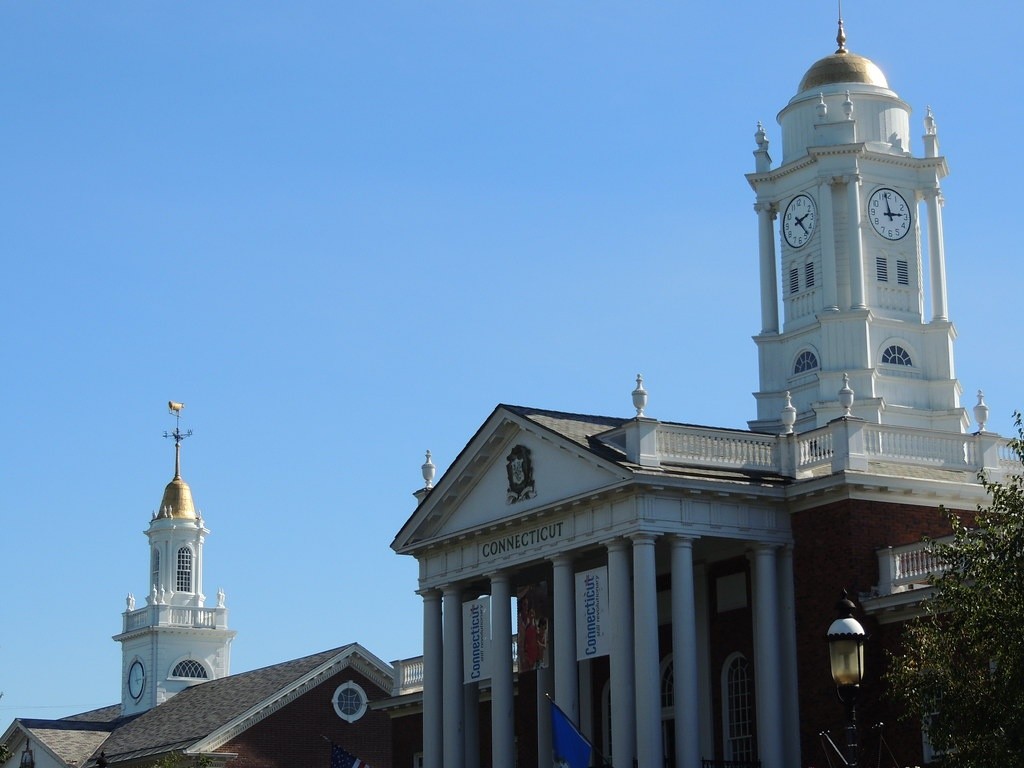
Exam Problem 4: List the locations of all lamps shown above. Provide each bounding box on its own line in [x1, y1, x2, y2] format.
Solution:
[865, 185, 913, 243]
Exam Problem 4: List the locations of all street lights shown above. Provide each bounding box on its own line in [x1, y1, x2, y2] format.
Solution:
[825, 589, 869, 768]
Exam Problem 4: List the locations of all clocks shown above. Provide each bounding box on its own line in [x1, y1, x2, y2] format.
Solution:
[126, 657, 146, 705]
[780, 192, 817, 250]
